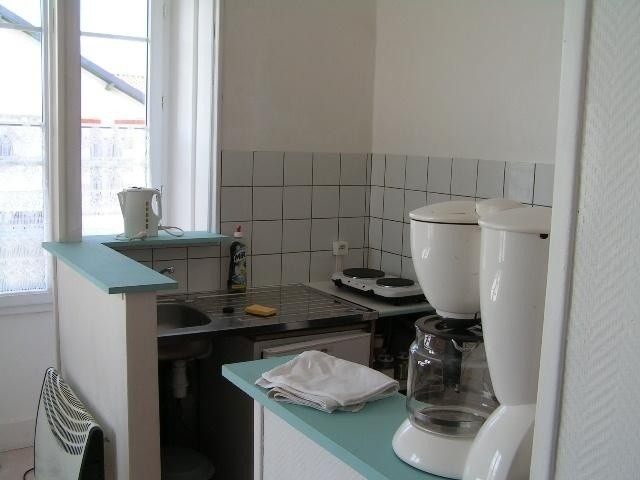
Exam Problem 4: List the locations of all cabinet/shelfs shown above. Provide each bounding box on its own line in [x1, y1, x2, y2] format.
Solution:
[255, 328, 373, 370]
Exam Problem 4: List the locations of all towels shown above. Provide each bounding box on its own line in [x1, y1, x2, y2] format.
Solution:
[254, 349, 400, 414]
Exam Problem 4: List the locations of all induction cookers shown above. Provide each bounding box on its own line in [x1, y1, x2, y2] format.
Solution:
[332, 266, 428, 301]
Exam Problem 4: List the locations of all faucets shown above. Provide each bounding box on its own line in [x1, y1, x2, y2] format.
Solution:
[158, 265, 176, 275]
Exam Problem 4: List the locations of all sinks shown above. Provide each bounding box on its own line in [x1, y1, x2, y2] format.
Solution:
[157, 296, 212, 332]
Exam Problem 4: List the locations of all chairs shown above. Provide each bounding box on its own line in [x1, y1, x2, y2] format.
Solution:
[32, 366, 106, 480]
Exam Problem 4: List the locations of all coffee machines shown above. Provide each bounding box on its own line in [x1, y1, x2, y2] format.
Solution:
[392, 200, 500, 477]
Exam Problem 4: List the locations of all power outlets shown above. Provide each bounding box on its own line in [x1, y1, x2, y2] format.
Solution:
[333, 241, 349, 258]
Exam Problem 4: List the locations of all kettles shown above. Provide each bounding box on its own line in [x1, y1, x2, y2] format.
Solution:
[115, 185, 184, 240]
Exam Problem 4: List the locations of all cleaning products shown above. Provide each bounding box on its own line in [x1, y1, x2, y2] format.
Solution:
[228, 225, 247, 293]
